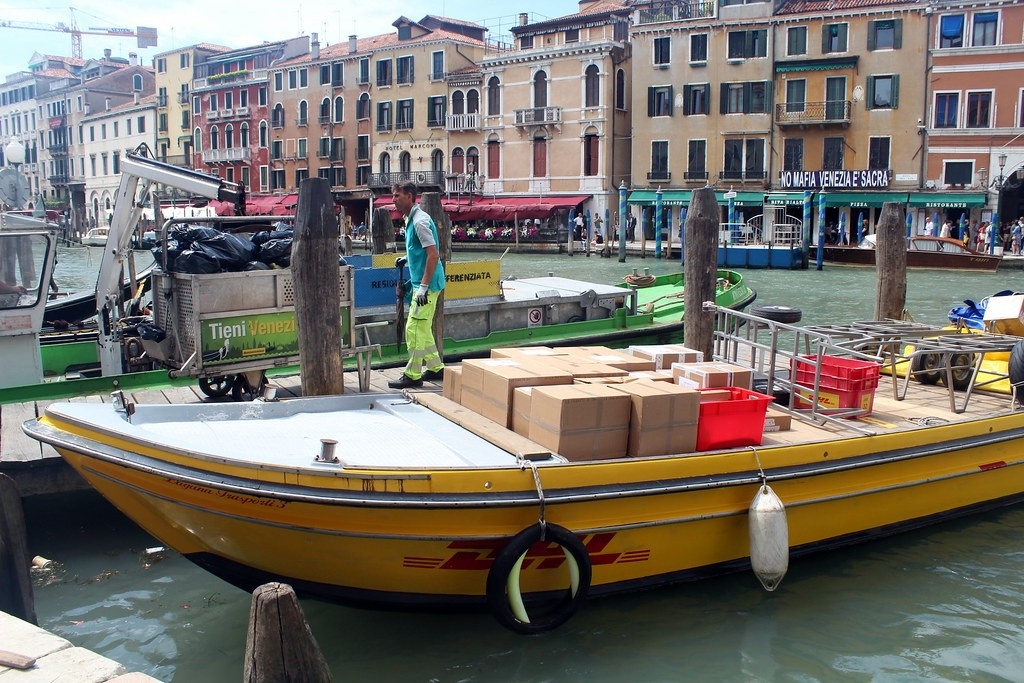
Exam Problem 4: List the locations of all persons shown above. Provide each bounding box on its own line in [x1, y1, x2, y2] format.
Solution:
[924, 217, 934, 237]
[0, 280, 26, 294]
[963, 216, 1024, 255]
[940, 220, 952, 245]
[82, 216, 96, 231]
[626, 213, 637, 243]
[593, 213, 603, 240]
[857, 219, 869, 238]
[351, 222, 368, 238]
[389, 183, 446, 389]
[824, 221, 848, 245]
[573, 213, 587, 241]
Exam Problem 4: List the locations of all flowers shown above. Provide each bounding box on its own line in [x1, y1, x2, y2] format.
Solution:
[207, 69, 249, 83]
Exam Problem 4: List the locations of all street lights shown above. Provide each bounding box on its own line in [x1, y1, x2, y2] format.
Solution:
[977, 152, 1024, 246]
[917, 7, 932, 192]
[457, 161, 486, 228]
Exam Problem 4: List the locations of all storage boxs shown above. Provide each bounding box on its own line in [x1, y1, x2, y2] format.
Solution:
[981, 295, 1024, 337]
[442, 344, 776, 461]
[763, 407, 792, 432]
[788, 356, 883, 420]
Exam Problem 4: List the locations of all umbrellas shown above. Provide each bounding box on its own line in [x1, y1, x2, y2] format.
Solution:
[395, 261, 405, 356]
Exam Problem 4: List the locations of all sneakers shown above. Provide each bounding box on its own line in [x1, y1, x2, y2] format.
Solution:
[421, 368, 444, 381]
[387, 374, 424, 389]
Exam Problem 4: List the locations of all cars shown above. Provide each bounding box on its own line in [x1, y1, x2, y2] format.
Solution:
[80, 226, 139, 249]
[142, 232, 156, 241]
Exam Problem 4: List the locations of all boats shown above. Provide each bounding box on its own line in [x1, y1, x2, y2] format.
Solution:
[21, 177, 1024, 635]
[808, 235, 1003, 273]
[0, 142, 757, 405]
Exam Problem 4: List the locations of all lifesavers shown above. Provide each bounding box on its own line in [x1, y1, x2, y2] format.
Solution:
[486, 522, 592, 637]
[1008, 339, 1024, 406]
[751, 380, 790, 406]
[911, 347, 976, 392]
[749, 303, 802, 324]
[125, 337, 145, 361]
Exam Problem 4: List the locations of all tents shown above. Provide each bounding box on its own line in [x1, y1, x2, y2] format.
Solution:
[105, 207, 218, 221]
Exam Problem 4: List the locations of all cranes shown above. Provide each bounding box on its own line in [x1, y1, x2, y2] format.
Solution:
[0, 7, 158, 59]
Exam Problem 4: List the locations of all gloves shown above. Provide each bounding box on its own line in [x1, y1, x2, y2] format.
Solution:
[395, 255, 407, 267]
[414, 284, 429, 307]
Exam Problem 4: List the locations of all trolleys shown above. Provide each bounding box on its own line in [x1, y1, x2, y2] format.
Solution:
[702, 300, 1024, 436]
[124, 214, 397, 402]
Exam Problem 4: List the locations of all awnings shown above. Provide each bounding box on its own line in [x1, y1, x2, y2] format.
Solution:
[628, 191, 763, 207]
[767, 193, 813, 205]
[814, 193, 987, 209]
[373, 195, 589, 210]
[203, 195, 298, 216]
